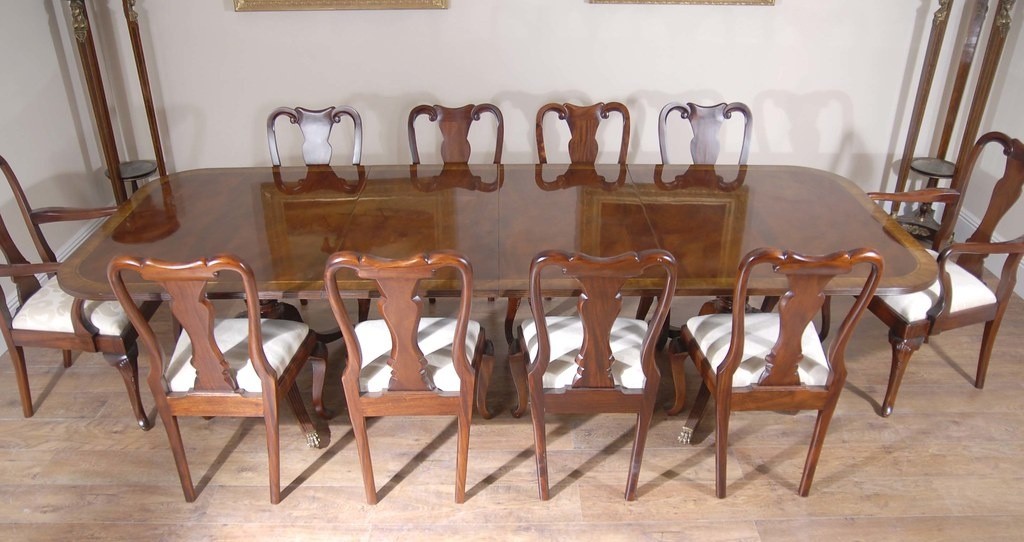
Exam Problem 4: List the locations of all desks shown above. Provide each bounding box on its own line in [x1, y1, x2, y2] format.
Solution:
[103, 160, 158, 192]
[59, 163, 939, 449]
[894, 155, 961, 249]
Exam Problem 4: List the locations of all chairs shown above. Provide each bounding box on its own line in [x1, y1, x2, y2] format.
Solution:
[0, 155, 181, 432]
[818, 131, 1024, 417]
[107, 102, 884, 500]
